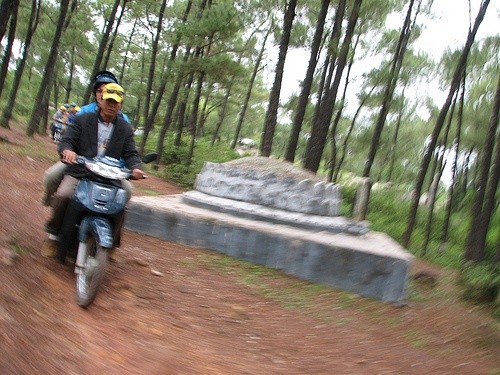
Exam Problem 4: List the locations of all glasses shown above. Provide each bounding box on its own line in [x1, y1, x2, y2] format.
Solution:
[103, 89, 124, 97]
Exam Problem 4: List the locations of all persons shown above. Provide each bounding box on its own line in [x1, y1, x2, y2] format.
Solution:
[39, 81, 145, 259]
[50, 101, 81, 146]
[42, 69, 133, 209]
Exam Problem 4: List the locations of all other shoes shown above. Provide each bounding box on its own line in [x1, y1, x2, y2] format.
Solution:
[107, 246, 120, 264]
[40, 233, 60, 260]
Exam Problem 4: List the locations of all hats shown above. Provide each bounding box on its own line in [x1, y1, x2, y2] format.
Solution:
[93, 75, 117, 90]
[102, 83, 125, 103]
[60, 101, 80, 112]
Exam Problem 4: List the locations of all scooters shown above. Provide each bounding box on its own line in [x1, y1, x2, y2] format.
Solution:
[55, 149, 159, 309]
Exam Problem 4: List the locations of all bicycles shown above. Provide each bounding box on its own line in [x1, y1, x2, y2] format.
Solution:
[50, 116, 65, 143]
